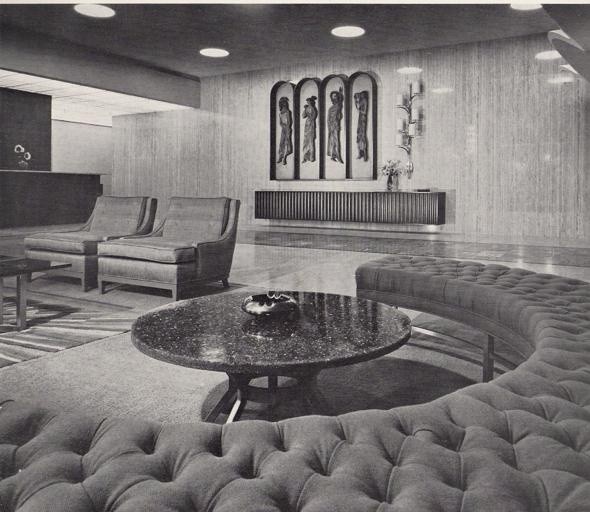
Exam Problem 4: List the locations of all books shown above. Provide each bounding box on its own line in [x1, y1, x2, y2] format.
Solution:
[0, 255, 51, 276]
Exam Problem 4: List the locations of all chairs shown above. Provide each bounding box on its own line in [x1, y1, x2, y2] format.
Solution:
[23, 193, 158, 292]
[96, 196, 241, 302]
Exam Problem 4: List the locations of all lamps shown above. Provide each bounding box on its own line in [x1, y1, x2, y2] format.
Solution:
[394, 79, 421, 179]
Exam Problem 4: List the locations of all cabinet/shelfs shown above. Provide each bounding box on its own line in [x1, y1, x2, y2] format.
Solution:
[254, 190, 446, 226]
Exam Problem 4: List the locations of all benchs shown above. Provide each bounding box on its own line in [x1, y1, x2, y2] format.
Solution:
[0, 253, 590, 512]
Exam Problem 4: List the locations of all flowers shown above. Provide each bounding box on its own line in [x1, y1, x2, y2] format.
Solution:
[13, 144, 32, 161]
[379, 159, 404, 176]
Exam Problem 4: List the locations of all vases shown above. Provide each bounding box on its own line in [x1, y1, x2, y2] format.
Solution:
[386, 175, 393, 191]
[16, 155, 29, 170]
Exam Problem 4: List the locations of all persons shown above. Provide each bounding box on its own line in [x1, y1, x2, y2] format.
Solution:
[354, 91, 368, 161]
[277, 97, 292, 164]
[301, 96, 318, 163]
[327, 87, 344, 164]
